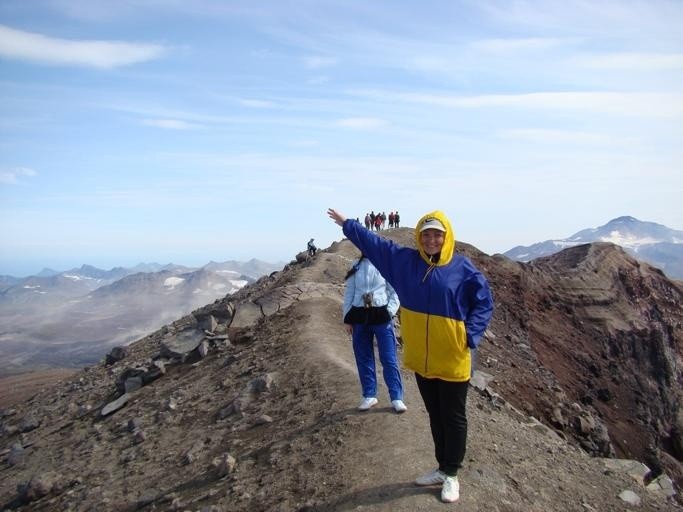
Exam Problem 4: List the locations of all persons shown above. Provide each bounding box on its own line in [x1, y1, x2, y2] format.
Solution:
[307, 238, 317, 256]
[326, 208, 494, 503]
[365, 211, 400, 231]
[343, 249, 409, 413]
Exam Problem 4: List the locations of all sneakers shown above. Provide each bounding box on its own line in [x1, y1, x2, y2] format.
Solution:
[359, 398, 377, 410]
[392, 400, 407, 412]
[415, 470, 449, 485]
[441, 477, 459, 502]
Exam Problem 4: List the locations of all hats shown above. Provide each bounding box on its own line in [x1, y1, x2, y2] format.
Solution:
[419, 218, 446, 233]
